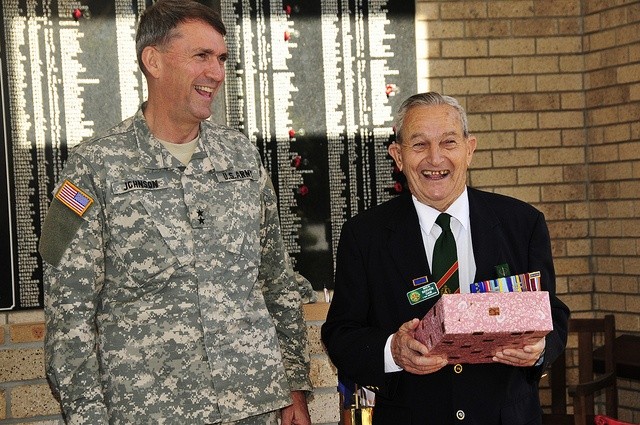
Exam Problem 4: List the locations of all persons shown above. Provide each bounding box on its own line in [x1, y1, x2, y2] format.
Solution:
[36, 0, 316, 425]
[321, 92, 572, 425]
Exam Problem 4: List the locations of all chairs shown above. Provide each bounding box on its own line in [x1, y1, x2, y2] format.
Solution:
[538, 314, 619, 424]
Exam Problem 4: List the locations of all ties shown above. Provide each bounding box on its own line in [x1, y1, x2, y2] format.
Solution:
[431, 213, 460, 296]
